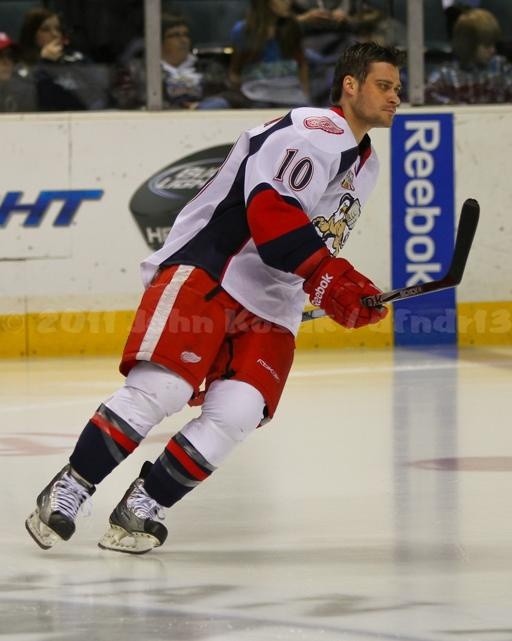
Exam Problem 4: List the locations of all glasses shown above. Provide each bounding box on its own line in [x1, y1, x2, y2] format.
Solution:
[162, 30, 192, 40]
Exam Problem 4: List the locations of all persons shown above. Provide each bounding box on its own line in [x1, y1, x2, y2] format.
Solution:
[33, 39, 405, 544]
[1, 1, 511, 115]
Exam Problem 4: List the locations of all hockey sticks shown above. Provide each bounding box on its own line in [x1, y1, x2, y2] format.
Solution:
[300, 200, 479, 323]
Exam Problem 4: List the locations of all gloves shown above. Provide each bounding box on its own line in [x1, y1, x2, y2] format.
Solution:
[301, 255, 389, 332]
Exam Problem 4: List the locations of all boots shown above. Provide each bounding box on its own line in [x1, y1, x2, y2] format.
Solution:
[35, 463, 98, 542]
[109, 460, 168, 547]
